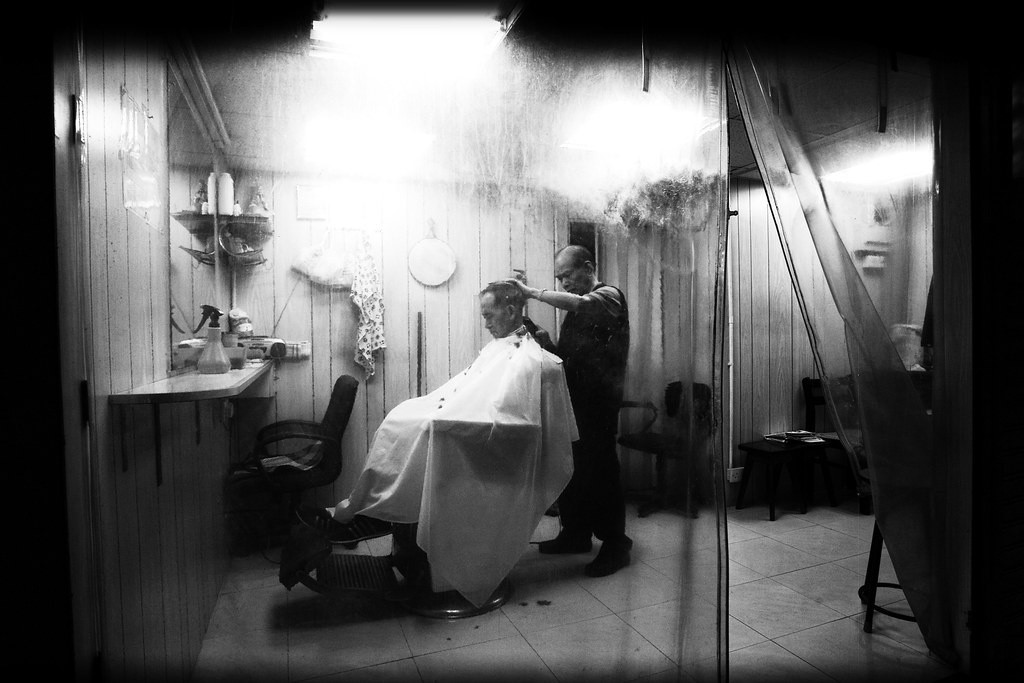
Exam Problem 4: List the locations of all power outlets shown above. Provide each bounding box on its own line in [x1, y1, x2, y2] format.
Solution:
[727, 467, 745, 483]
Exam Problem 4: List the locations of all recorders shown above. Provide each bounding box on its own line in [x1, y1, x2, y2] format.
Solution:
[236, 279, 301, 360]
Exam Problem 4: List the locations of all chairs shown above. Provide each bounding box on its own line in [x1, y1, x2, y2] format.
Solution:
[616, 381, 712, 518]
[222, 375, 359, 562]
[277, 365, 563, 621]
[802, 377, 871, 516]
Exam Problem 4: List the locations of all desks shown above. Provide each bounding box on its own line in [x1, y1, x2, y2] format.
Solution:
[108, 359, 276, 405]
[737, 440, 834, 520]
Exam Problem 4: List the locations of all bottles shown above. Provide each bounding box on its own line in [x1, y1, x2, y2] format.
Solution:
[207, 172, 216, 214]
[219, 173, 234, 216]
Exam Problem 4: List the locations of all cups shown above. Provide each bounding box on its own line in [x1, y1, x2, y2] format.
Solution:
[224, 348, 248, 369]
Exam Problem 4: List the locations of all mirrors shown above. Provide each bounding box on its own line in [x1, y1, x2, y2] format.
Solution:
[164, 64, 218, 377]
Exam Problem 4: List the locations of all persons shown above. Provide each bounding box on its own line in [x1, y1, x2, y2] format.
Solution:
[505, 245, 633, 577]
[297, 279, 526, 546]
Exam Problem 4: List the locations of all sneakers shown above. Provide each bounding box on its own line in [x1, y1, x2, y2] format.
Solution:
[295, 505, 393, 544]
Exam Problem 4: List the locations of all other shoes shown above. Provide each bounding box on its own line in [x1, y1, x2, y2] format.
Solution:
[539, 532, 593, 554]
[585, 541, 630, 577]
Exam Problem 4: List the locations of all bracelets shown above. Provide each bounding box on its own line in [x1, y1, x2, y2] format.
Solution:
[537, 288, 547, 302]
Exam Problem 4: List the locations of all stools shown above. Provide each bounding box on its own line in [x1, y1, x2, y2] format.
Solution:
[860, 486, 930, 636]
